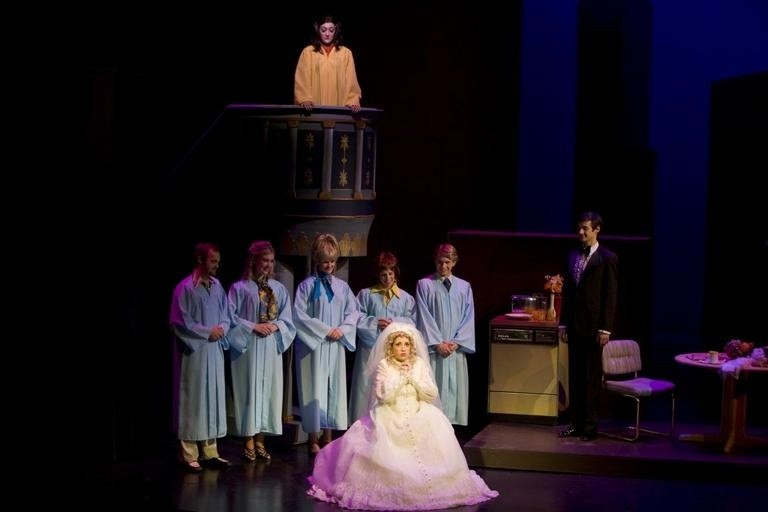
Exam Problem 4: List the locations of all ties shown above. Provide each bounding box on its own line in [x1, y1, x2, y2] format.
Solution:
[443, 279, 450, 291]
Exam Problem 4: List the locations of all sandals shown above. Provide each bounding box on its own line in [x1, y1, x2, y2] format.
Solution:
[245, 446, 271, 461]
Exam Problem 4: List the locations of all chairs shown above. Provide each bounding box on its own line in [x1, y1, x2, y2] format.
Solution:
[600, 340, 675, 442]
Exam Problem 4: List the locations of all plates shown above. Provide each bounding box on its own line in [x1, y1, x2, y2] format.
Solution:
[703, 359, 723, 365]
[503, 311, 533, 319]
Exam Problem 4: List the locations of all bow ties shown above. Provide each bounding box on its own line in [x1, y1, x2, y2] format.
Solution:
[578, 246, 591, 256]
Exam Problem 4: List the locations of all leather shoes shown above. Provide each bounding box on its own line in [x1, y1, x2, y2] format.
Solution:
[559, 428, 577, 436]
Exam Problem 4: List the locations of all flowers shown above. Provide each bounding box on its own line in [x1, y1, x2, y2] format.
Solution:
[543, 273, 563, 292]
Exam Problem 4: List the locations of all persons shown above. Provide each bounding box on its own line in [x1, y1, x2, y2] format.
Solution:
[304, 317, 500, 511]
[169, 243, 233, 472]
[347, 251, 419, 430]
[227, 242, 297, 461]
[294, 16, 363, 114]
[292, 234, 358, 455]
[415, 244, 476, 426]
[559, 213, 620, 437]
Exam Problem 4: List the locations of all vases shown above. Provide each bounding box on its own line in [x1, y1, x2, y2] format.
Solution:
[547, 295, 556, 318]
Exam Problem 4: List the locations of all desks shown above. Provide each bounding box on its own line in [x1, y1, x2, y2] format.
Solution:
[487, 308, 560, 427]
[674, 351, 768, 453]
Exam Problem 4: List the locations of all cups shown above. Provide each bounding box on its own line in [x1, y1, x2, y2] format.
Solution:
[709, 351, 718, 363]
